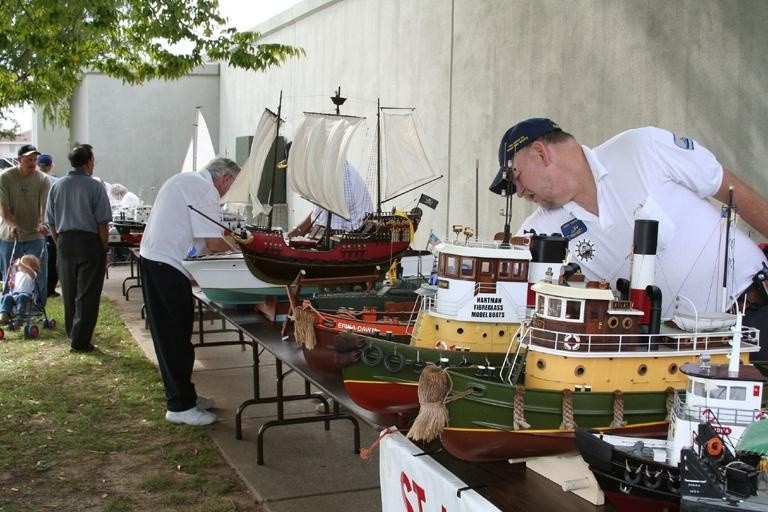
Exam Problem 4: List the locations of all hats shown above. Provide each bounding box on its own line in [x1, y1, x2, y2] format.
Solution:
[17, 145, 42, 156]
[489, 117, 561, 194]
[38, 154, 53, 166]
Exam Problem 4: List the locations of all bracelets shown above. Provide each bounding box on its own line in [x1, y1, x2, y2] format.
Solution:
[296, 226, 305, 237]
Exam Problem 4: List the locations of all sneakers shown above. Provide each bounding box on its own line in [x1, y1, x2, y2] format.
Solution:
[192, 394, 215, 408]
[69, 344, 100, 355]
[166, 406, 218, 426]
[13, 314, 23, 322]
[1, 313, 8, 323]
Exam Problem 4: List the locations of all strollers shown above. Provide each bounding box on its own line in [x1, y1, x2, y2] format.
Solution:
[0, 224, 55, 338]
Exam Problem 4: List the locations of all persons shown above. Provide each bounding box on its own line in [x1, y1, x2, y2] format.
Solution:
[138, 156, 242, 427]
[487, 117, 768, 378]
[277, 140, 374, 241]
[0, 143, 141, 355]
